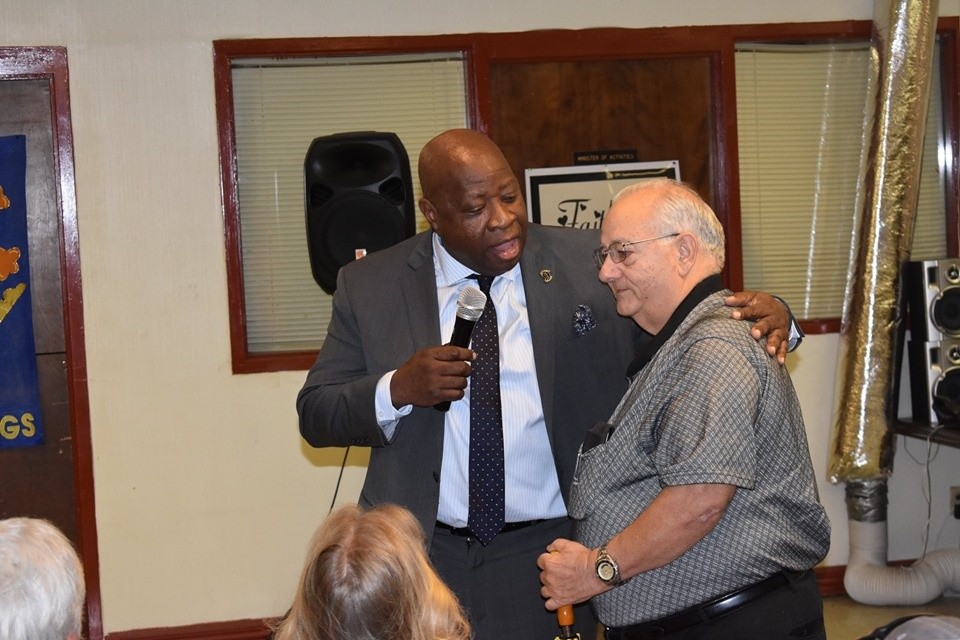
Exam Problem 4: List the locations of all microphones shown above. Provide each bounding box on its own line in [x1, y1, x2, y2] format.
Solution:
[432, 287, 486, 411]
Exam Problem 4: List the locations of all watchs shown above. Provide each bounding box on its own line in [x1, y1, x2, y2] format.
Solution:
[594, 542, 632, 586]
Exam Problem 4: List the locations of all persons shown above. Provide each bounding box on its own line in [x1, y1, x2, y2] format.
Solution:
[0, 518, 86, 640]
[538, 177, 828, 640]
[279, 502, 471, 639]
[297, 129, 805, 640]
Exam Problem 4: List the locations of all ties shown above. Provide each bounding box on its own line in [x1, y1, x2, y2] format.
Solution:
[464, 274, 505, 546]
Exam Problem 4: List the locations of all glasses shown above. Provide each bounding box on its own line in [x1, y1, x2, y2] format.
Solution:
[592, 232, 681, 271]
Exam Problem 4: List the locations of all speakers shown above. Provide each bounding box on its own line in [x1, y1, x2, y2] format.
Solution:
[905, 256, 960, 425]
[304, 130, 417, 296]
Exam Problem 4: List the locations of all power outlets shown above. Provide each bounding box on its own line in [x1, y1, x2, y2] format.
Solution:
[949, 485, 960, 516]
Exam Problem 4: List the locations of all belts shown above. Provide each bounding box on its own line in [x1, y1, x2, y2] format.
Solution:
[435, 518, 544, 538]
[604, 568, 810, 640]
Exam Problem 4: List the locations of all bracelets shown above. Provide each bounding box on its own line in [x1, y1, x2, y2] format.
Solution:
[773, 296, 804, 353]
